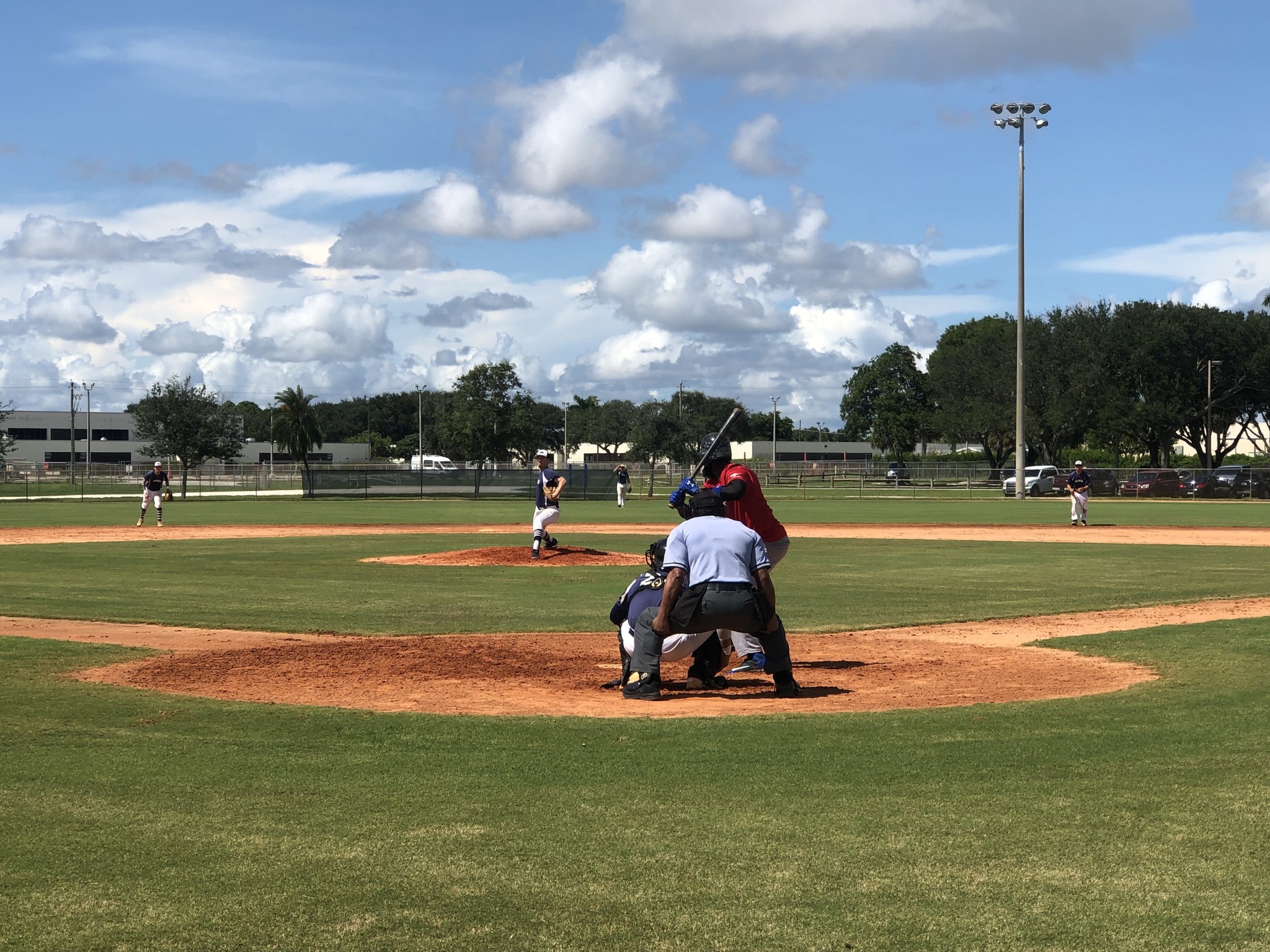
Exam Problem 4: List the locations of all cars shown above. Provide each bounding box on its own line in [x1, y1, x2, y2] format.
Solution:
[1179, 475, 1239, 499]
[1174, 470, 1193, 479]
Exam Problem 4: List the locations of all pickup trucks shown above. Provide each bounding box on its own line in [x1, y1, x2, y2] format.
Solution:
[1003, 465, 1070, 497]
[1052, 468, 1119, 497]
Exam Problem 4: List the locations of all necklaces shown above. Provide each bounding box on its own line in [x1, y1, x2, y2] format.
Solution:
[155, 472, 161, 478]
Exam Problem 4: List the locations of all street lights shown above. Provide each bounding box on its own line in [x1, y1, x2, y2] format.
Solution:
[414, 383, 430, 499]
[816, 422, 825, 442]
[562, 402, 570, 469]
[769, 395, 780, 482]
[1206, 360, 1224, 473]
[82, 382, 95, 478]
[268, 402, 279, 479]
[992, 98, 1063, 493]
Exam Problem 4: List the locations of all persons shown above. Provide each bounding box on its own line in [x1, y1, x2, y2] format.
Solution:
[623, 488, 801, 701]
[669, 432, 790, 674]
[532, 449, 566, 560]
[136, 461, 174, 527]
[613, 464, 631, 509]
[1066, 460, 1091, 526]
[610, 539, 727, 690]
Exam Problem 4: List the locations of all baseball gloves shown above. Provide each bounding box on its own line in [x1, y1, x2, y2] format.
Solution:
[1076, 487, 1086, 494]
[542, 486, 561, 501]
[163, 491, 173, 502]
[627, 488, 632, 493]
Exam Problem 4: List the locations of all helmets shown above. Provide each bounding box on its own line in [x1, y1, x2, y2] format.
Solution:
[697, 433, 732, 466]
[645, 539, 669, 570]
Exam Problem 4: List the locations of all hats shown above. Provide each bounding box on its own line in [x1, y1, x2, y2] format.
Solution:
[535, 449, 550, 459]
[620, 464, 625, 467]
[690, 490, 724, 509]
[1075, 461, 1083, 467]
[153, 462, 162, 467]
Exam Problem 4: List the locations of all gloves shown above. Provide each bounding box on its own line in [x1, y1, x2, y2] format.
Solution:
[670, 476, 700, 509]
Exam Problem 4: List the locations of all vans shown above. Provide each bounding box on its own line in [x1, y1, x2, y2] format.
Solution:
[411, 453, 462, 481]
[1208, 465, 1252, 475]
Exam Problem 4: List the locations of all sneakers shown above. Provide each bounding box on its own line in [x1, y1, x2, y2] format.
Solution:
[776, 679, 801, 697]
[623, 676, 662, 701]
[532, 549, 540, 560]
[687, 675, 726, 690]
[544, 538, 559, 548]
[729, 656, 765, 674]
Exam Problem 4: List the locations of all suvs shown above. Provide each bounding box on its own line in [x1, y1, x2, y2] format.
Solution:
[885, 461, 912, 484]
[1232, 468, 1270, 499]
[1121, 470, 1182, 499]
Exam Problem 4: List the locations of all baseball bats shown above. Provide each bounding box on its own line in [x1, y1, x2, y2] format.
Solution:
[668, 408, 742, 509]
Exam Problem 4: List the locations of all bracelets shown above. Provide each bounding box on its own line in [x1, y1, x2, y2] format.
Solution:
[143, 490, 147, 494]
[166, 486, 170, 489]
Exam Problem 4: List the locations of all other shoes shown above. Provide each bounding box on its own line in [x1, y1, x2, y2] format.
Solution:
[1071, 521, 1078, 526]
[618, 505, 624, 508]
[1081, 522, 1087, 526]
[157, 521, 163, 527]
[137, 518, 144, 527]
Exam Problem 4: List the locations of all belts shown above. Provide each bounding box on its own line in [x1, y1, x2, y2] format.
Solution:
[707, 582, 743, 591]
